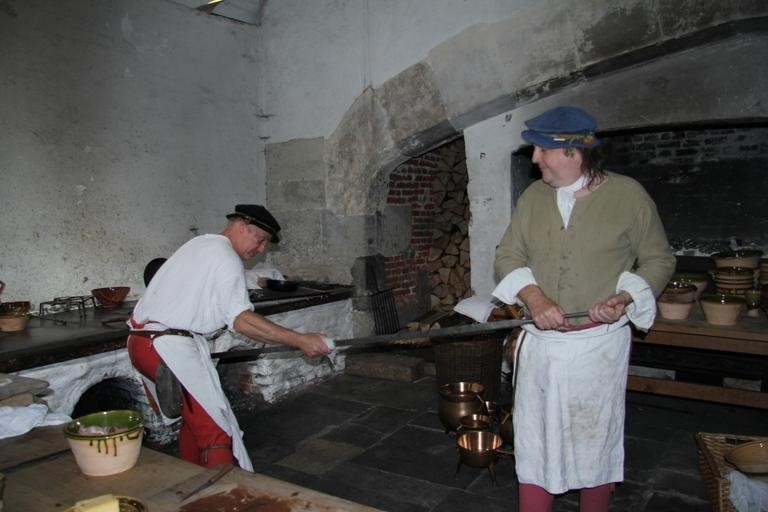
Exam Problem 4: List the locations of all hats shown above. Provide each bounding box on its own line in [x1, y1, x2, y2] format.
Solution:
[522, 105, 602, 149]
[225, 204, 281, 244]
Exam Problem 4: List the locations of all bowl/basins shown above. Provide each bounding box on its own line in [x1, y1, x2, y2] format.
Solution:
[64, 409, 146, 477]
[728, 437, 767, 478]
[0, 314, 29, 333]
[655, 242, 768, 325]
[92, 287, 130, 311]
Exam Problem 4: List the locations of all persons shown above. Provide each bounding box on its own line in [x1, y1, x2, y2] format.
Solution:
[127, 205, 333, 468]
[495, 107, 677, 512]
